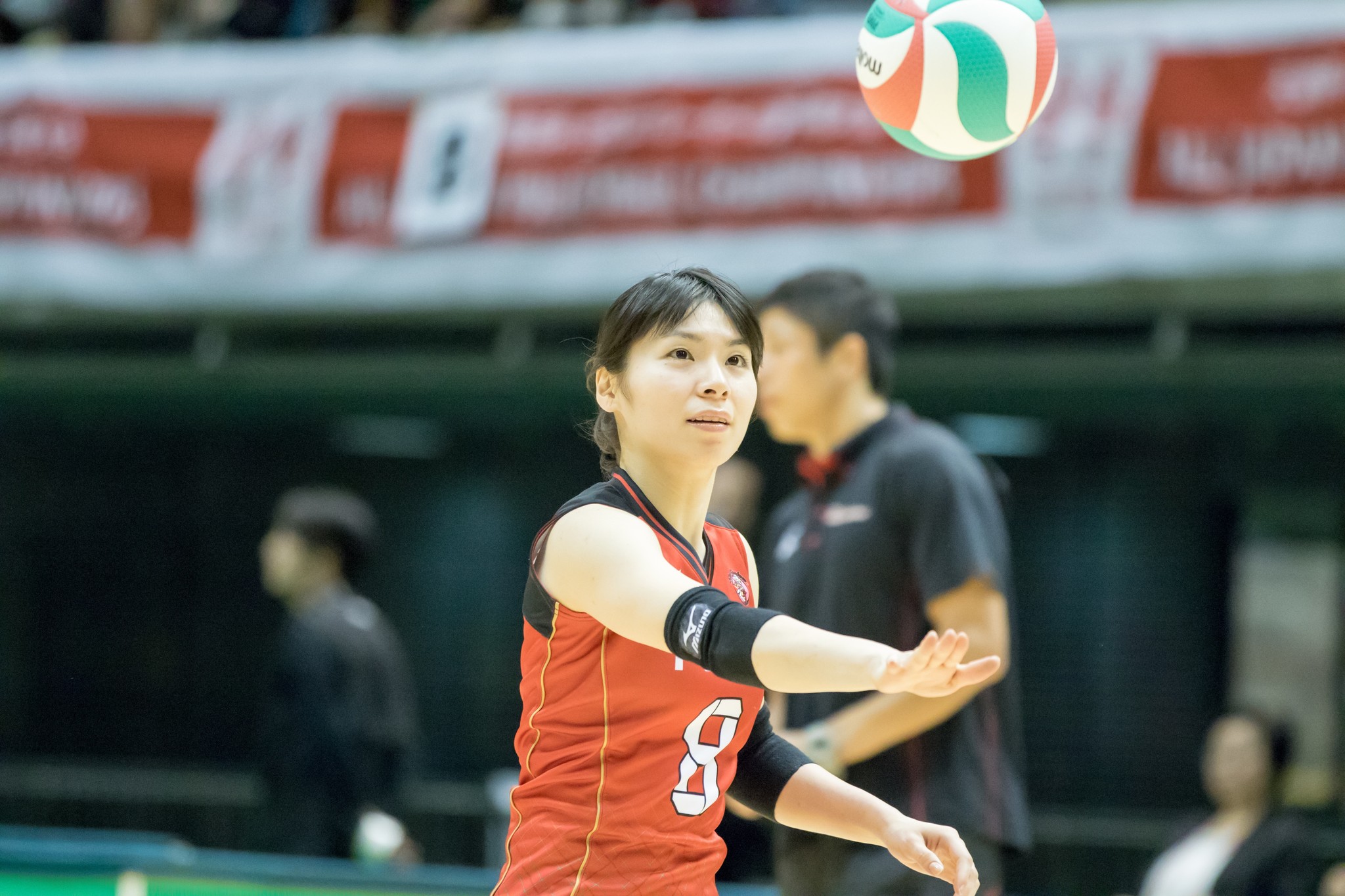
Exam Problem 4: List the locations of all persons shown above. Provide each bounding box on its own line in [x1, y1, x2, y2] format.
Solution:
[247, 484, 429, 868]
[486, 268, 1000, 896]
[709, 267, 1039, 896]
[1138, 704, 1345, 896]
[1, 1, 693, 47]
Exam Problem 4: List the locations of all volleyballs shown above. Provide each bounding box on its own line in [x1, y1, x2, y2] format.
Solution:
[854, 0, 1059, 162]
